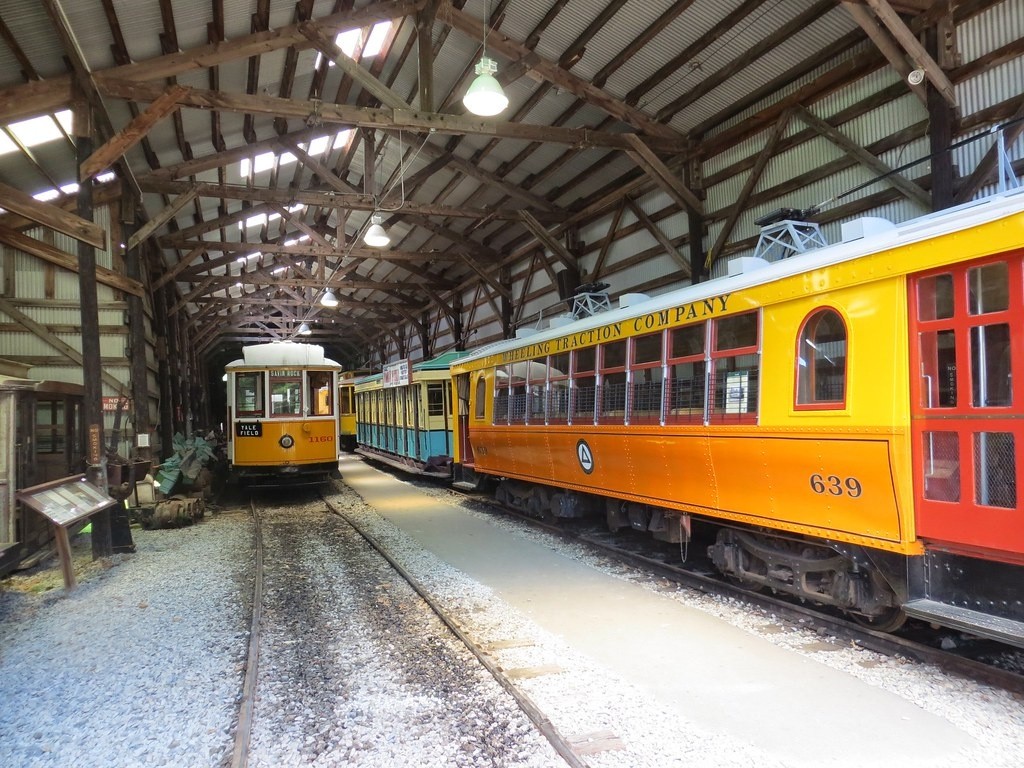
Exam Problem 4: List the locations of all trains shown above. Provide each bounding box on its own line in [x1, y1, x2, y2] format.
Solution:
[316, 166, 1024, 651]
[222, 339, 344, 492]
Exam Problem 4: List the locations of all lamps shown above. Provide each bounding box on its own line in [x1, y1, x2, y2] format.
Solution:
[908, 66, 926, 85]
[297, 324, 312, 335]
[364, 197, 391, 247]
[320, 256, 339, 307]
[462, 0, 510, 117]
